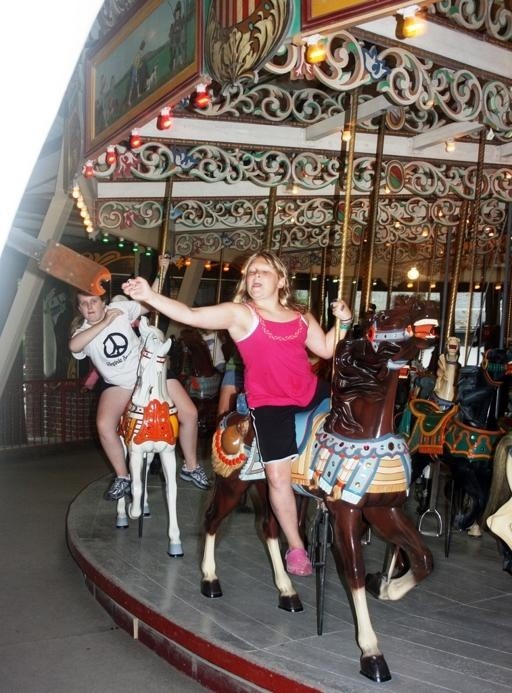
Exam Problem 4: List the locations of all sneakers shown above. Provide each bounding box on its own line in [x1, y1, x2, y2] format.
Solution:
[105, 473, 131, 500]
[284, 547, 313, 577]
[179, 463, 211, 491]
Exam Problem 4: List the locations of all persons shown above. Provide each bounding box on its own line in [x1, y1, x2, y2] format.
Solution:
[92, 0, 192, 140]
[68, 252, 211, 499]
[121, 249, 354, 578]
[114, 301, 511, 683]
